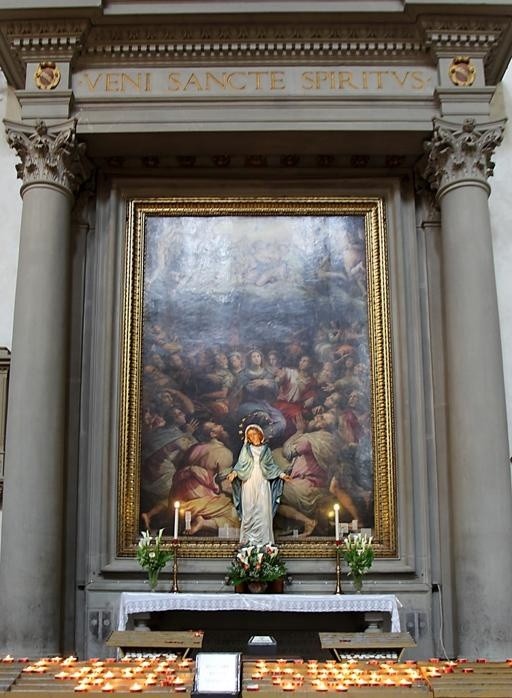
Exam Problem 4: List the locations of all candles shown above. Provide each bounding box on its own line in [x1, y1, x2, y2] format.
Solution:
[174, 508, 179, 538]
[335, 511, 339, 541]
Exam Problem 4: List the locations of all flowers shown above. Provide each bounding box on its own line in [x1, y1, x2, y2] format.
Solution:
[219, 539, 287, 586]
[338, 531, 374, 588]
[131, 528, 175, 585]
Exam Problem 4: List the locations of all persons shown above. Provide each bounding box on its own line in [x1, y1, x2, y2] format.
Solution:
[224, 422, 294, 549]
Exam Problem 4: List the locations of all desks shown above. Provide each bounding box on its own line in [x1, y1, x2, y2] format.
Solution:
[319, 632, 416, 661]
[105, 631, 205, 658]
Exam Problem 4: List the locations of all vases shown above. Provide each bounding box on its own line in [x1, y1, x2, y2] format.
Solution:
[352, 574, 363, 592]
[247, 581, 268, 592]
[146, 566, 159, 592]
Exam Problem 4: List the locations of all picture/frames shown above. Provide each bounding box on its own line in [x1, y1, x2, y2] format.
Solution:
[116, 195, 398, 560]
